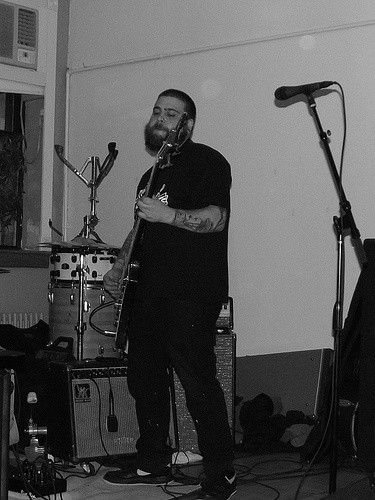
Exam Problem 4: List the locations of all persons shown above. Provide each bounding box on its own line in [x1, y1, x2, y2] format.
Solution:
[102, 89, 237, 500]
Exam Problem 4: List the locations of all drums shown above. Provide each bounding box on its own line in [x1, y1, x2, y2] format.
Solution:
[48, 282, 122, 361]
[48, 250, 118, 284]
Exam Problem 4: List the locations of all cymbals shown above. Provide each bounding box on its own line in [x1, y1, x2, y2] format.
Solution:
[38, 237, 117, 249]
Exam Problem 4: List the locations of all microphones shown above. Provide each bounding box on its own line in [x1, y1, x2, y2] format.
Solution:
[274, 81, 333, 100]
[106, 390, 118, 433]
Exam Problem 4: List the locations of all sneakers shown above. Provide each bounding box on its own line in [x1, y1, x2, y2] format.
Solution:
[103, 461, 174, 487]
[198, 470, 239, 500]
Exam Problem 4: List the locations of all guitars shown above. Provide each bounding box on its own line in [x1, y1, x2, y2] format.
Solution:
[113, 130, 177, 349]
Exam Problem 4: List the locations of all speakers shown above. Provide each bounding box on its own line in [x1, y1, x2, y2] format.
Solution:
[327, 237, 375, 470]
[46, 367, 179, 466]
[165, 328, 238, 462]
